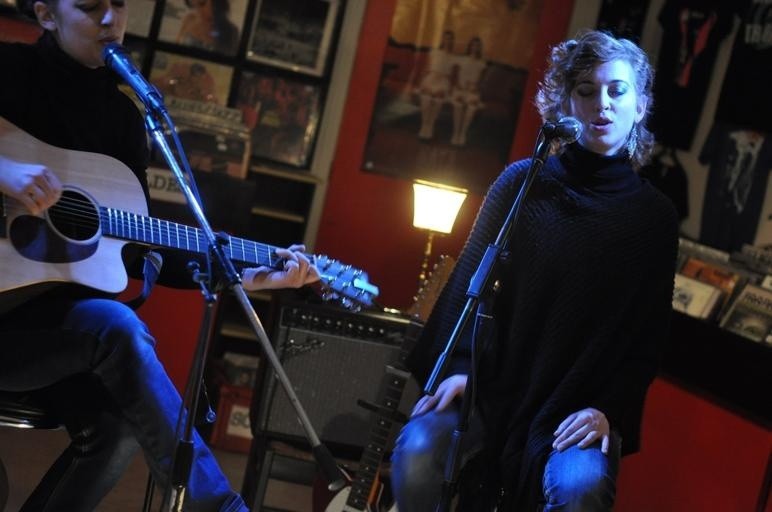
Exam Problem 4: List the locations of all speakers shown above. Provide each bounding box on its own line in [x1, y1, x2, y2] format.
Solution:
[250, 291, 426, 460]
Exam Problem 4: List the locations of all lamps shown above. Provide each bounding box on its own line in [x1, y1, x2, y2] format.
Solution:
[403, 178, 469, 319]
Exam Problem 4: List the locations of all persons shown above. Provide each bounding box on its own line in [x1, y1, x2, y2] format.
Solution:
[0, 0, 319, 512]
[455, 36, 486, 148]
[419, 30, 457, 139]
[390, 33, 690, 511]
[158, 1, 314, 163]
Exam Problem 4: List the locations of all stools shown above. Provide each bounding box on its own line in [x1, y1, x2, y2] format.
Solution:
[1, 403, 60, 438]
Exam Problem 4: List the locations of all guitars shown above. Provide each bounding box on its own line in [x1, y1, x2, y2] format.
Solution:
[0, 113, 379, 311]
[312, 255, 457, 511]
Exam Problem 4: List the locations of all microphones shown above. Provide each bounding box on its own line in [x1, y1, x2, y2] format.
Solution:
[543, 116, 583, 144]
[102, 41, 164, 116]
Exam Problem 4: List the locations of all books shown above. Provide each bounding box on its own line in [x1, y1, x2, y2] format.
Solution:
[672, 234, 771, 350]
